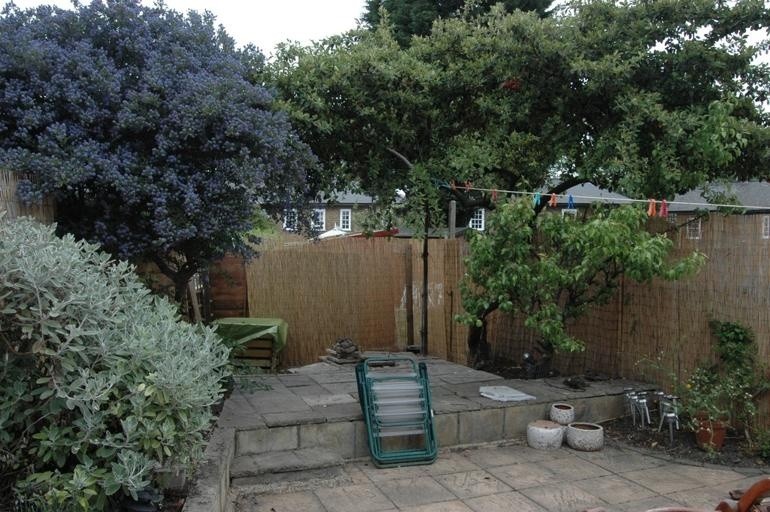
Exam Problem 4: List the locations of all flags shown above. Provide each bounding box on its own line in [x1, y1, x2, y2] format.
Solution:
[526, 402, 606, 451]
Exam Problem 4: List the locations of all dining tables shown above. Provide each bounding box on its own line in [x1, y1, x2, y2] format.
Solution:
[645, 323, 770, 464]
